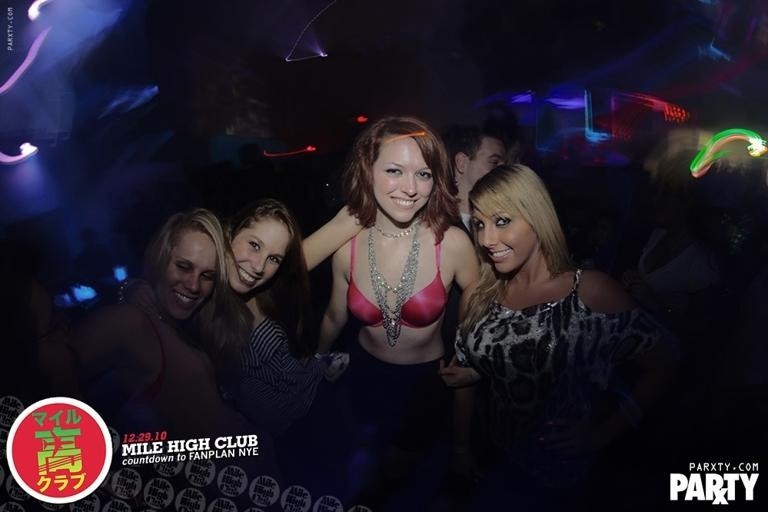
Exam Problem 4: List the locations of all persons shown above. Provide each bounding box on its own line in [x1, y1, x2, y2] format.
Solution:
[25, 114, 723, 512]
